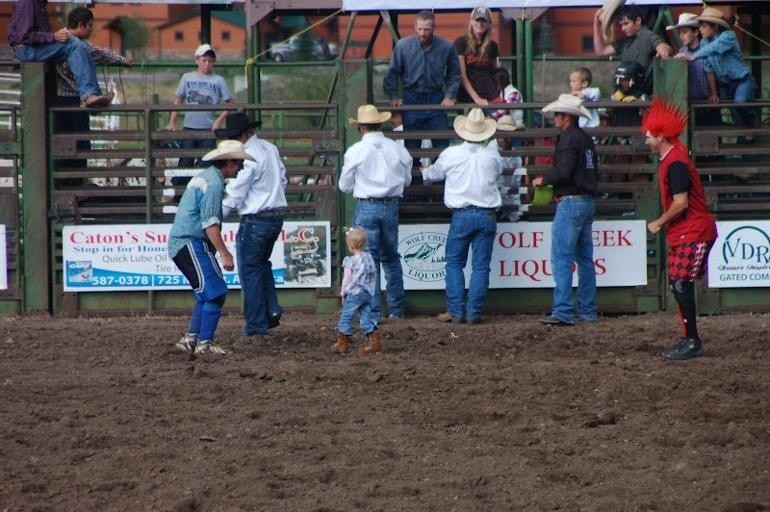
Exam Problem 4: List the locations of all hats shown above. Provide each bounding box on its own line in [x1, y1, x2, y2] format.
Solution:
[666, 12, 701, 30]
[697, 7, 731, 29]
[598, 0, 626, 39]
[453, 107, 497, 142]
[194, 43, 215, 56]
[348, 105, 392, 128]
[201, 140, 256, 162]
[214, 113, 262, 138]
[496, 115, 527, 131]
[470, 6, 492, 21]
[542, 93, 592, 120]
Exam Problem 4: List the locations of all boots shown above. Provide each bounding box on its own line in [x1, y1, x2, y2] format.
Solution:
[362, 328, 381, 353]
[331, 331, 348, 355]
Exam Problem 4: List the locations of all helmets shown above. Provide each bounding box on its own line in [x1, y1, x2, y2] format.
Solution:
[612, 61, 643, 95]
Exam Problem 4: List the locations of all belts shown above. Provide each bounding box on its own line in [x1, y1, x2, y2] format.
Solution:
[360, 198, 393, 201]
[555, 194, 593, 202]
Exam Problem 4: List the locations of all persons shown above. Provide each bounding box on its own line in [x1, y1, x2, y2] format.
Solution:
[533, 92, 601, 326]
[487, 65, 524, 132]
[383, 11, 462, 189]
[591, 0, 671, 85]
[421, 108, 504, 324]
[666, 12, 716, 102]
[389, 97, 434, 169]
[338, 104, 414, 320]
[167, 139, 259, 357]
[595, 108, 617, 145]
[333, 229, 383, 354]
[454, 7, 509, 105]
[566, 67, 601, 143]
[165, 43, 235, 202]
[673, 8, 759, 149]
[489, 114, 522, 220]
[213, 113, 288, 336]
[603, 60, 649, 127]
[52, 7, 132, 187]
[642, 98, 719, 362]
[7, 0, 116, 117]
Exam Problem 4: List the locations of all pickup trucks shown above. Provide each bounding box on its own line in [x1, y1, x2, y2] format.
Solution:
[270, 35, 339, 62]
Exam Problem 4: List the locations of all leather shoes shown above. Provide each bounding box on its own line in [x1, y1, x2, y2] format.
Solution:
[661, 337, 702, 359]
[80, 92, 114, 115]
[267, 312, 281, 328]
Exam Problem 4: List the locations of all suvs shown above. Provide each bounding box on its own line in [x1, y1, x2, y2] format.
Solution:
[286, 245, 326, 281]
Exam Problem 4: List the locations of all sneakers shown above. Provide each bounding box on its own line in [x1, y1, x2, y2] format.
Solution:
[194, 340, 227, 357]
[438, 313, 462, 324]
[175, 333, 199, 354]
[539, 315, 561, 324]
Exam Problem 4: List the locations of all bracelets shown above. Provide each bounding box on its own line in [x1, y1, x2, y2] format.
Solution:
[217, 251, 230, 258]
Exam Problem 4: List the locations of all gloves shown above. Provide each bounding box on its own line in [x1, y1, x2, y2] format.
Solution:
[614, 90, 625, 101]
[622, 95, 637, 103]
